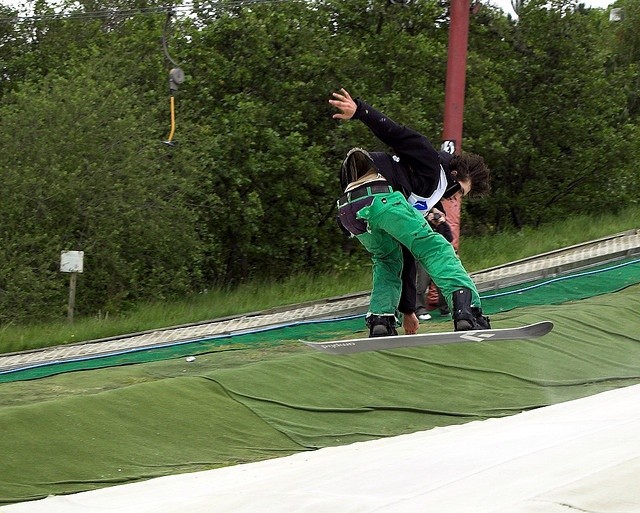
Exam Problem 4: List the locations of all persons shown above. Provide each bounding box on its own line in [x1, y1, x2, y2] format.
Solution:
[415, 201, 453, 320]
[329, 88, 492, 338]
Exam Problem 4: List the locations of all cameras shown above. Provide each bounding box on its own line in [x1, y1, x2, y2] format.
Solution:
[434, 212, 441, 220]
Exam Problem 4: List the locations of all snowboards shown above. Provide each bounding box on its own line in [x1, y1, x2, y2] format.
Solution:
[297, 320, 554, 354]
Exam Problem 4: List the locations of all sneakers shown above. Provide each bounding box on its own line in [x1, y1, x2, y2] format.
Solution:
[365, 310, 399, 336]
[453, 307, 491, 330]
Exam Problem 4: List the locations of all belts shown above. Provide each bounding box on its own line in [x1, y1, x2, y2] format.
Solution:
[338, 185, 391, 205]
